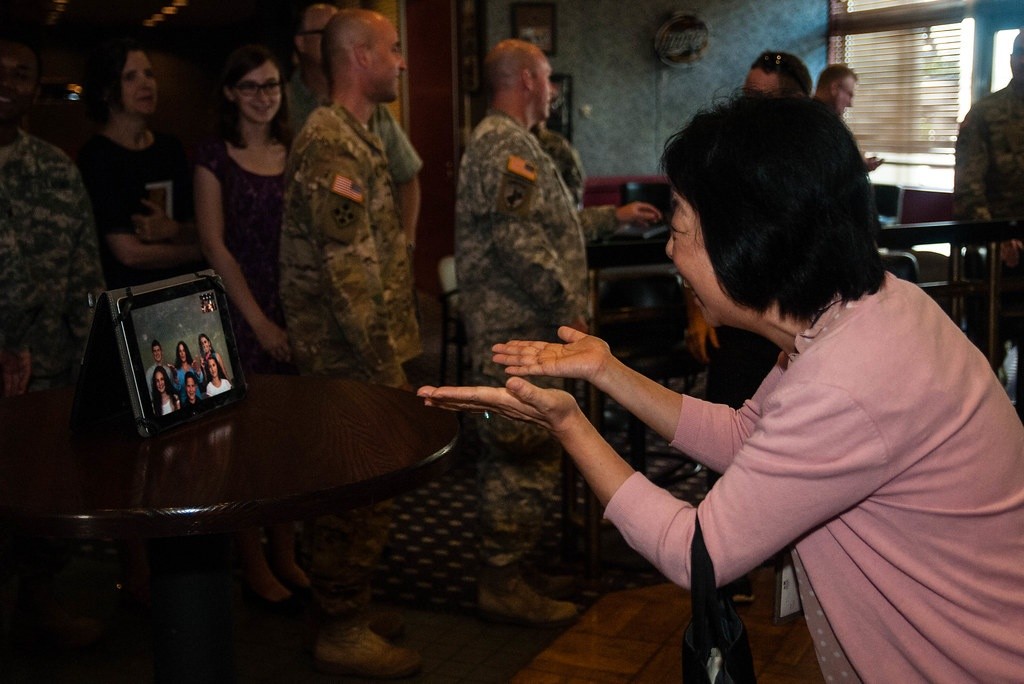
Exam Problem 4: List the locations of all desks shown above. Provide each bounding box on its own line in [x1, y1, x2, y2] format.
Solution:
[0, 373, 461, 684]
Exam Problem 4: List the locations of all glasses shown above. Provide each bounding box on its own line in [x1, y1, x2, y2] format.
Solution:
[234, 80, 281, 96]
[757, 52, 810, 95]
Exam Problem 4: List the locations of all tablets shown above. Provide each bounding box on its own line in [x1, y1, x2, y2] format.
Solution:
[117, 274, 248, 433]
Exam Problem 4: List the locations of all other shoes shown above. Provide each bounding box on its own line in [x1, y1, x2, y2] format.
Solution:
[470, 582, 579, 630]
[310, 627, 424, 680]
[531, 576, 580, 598]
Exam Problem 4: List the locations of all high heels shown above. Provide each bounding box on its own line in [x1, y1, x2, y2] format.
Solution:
[244, 578, 303, 606]
[274, 569, 324, 597]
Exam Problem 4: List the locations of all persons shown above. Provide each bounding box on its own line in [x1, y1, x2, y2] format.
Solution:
[183, 372, 201, 407]
[686, 51, 813, 490]
[151, 366, 181, 416]
[173, 340, 204, 404]
[198, 333, 229, 383]
[815, 64, 885, 172]
[193, 43, 315, 616]
[415, 86, 1024, 684]
[277, 9, 423, 679]
[145, 339, 179, 400]
[454, 38, 662, 629]
[273, 3, 423, 263]
[0, 35, 107, 649]
[75, 38, 210, 621]
[528, 83, 586, 212]
[205, 357, 232, 398]
[954, 30, 1024, 427]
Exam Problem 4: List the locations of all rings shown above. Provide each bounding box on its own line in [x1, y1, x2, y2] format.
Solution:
[484, 410, 489, 418]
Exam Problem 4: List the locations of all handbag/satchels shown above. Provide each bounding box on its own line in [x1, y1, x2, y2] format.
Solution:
[682, 514, 756, 684]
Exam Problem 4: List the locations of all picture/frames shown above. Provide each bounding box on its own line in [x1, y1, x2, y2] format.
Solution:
[511, 1, 557, 56]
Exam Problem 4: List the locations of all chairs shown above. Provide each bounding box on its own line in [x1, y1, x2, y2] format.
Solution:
[439, 176, 1024, 566]
